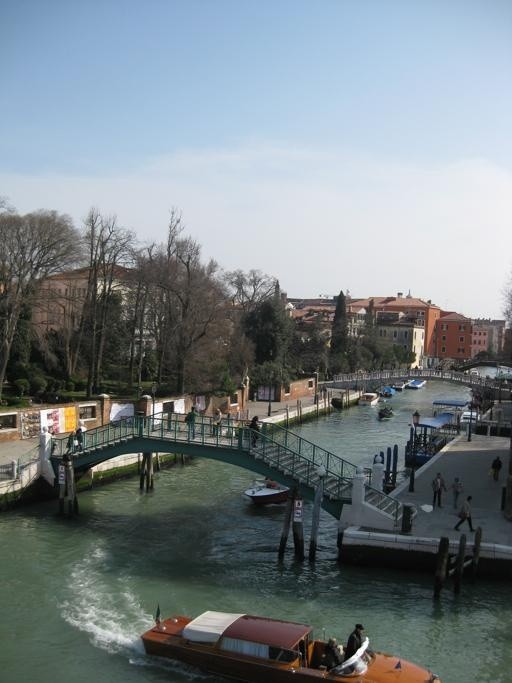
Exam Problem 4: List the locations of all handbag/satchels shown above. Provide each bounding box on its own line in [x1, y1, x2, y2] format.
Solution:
[458, 511, 467, 520]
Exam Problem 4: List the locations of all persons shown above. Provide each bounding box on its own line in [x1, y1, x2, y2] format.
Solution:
[255, 476, 279, 489]
[491, 456, 504, 482]
[448, 476, 464, 508]
[405, 361, 459, 379]
[49, 427, 84, 454]
[210, 408, 222, 439]
[454, 496, 476, 533]
[249, 415, 258, 448]
[431, 472, 446, 509]
[187, 406, 199, 440]
[344, 623, 366, 672]
[383, 406, 392, 414]
[323, 636, 343, 671]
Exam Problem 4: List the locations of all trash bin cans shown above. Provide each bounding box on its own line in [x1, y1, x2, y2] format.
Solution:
[314, 396, 317, 404]
[328, 391, 332, 398]
[399, 502, 415, 534]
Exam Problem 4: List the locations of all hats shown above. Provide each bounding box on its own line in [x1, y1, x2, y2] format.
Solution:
[356, 623, 364, 630]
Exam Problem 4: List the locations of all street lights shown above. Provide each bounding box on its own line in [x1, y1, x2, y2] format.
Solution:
[408, 410, 421, 493]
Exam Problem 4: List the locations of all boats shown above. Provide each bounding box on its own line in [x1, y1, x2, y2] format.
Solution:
[378, 408, 393, 418]
[358, 376, 428, 407]
[140, 608, 441, 683]
[243, 475, 292, 504]
